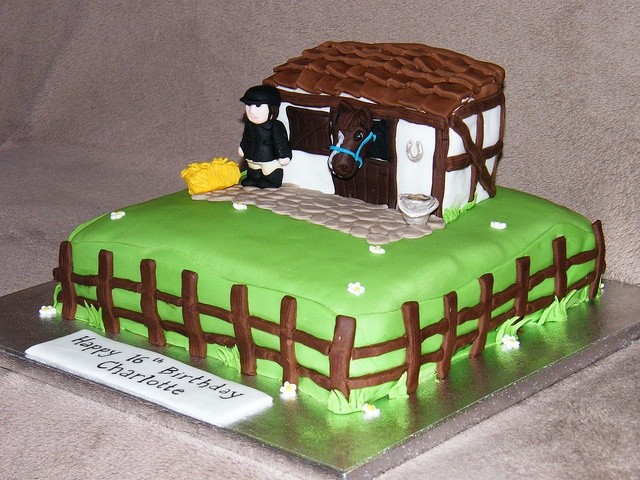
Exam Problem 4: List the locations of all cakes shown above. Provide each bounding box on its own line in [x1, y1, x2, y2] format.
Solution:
[37, 42, 606, 420]
